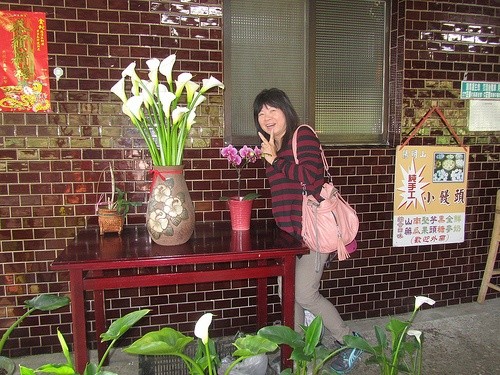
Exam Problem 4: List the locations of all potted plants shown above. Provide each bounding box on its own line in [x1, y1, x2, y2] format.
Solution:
[97, 162, 143, 235]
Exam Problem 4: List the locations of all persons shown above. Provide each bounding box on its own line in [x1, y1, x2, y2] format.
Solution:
[253, 87, 368, 373]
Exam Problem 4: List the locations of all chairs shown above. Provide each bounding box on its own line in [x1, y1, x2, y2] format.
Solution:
[477, 190, 500, 304]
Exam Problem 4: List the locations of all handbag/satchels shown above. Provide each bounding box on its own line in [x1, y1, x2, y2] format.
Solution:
[300, 182, 359, 261]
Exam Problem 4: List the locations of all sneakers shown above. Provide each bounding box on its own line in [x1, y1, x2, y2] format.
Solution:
[329, 330, 367, 375]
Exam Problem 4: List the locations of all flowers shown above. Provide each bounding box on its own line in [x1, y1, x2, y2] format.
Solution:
[110, 54, 225, 166]
[219, 144, 272, 201]
[0, 294, 435, 375]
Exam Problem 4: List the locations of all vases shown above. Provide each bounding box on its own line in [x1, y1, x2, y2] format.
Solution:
[146, 166, 195, 246]
[0, 356, 16, 375]
[227, 197, 253, 230]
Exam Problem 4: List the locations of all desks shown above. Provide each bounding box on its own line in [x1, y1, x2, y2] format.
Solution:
[50, 222, 309, 375]
